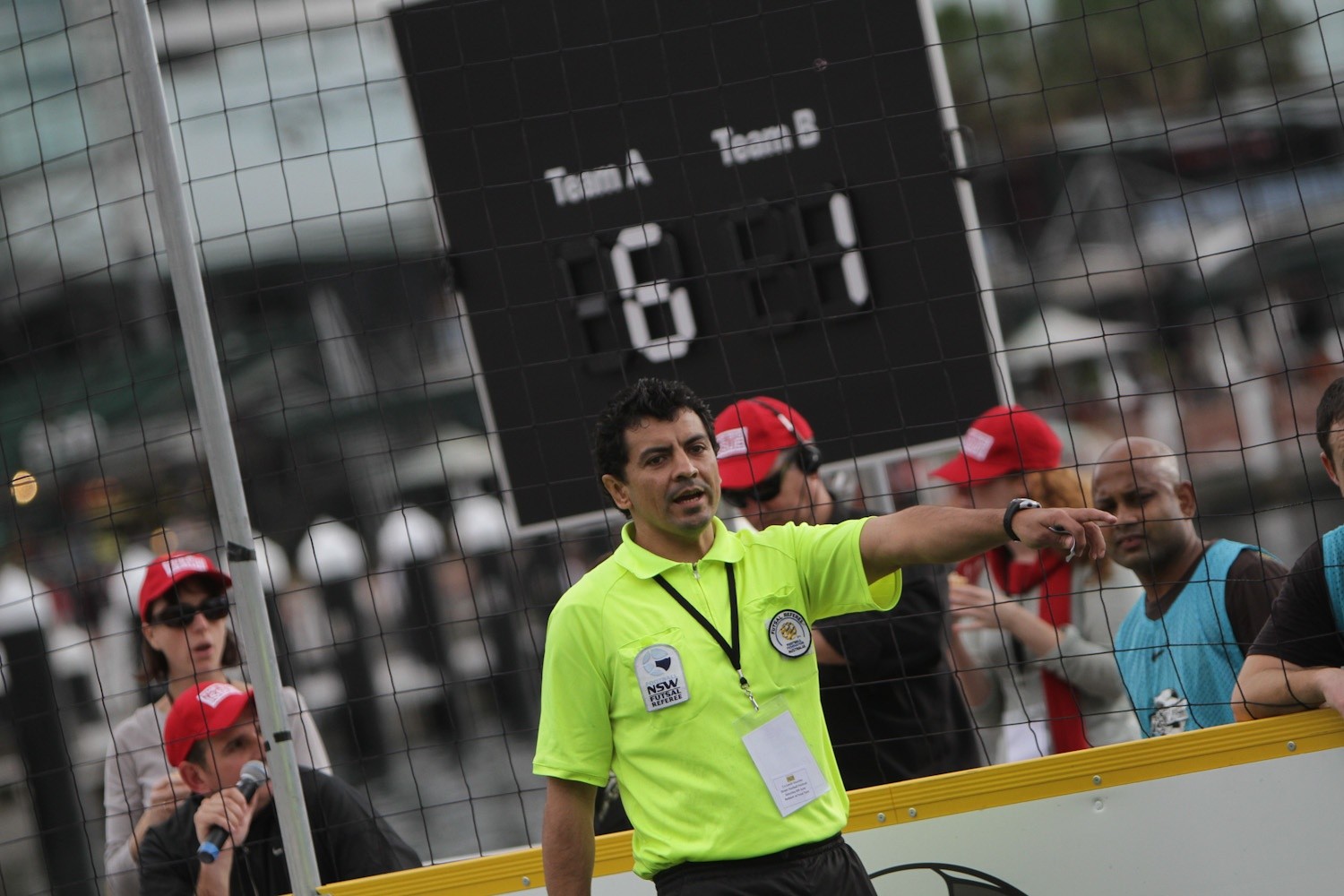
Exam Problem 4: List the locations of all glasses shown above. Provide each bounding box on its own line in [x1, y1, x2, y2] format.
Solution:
[721, 452, 801, 505]
[144, 594, 232, 634]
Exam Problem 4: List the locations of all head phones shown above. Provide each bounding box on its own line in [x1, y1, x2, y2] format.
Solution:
[745, 397, 822, 477]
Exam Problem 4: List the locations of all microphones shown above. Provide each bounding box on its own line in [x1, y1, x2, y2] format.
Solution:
[198, 760, 267, 863]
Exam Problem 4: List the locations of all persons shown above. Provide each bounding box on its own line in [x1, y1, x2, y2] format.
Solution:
[911, 407, 1147, 767]
[106, 554, 333, 895]
[139, 682, 424, 896]
[0, 411, 203, 825]
[694, 397, 983, 797]
[1089, 435, 1298, 742]
[1230, 374, 1344, 725]
[528, 377, 1124, 896]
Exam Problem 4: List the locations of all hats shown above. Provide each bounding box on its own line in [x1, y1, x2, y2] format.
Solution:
[930, 404, 1062, 486]
[712, 399, 818, 486]
[140, 551, 232, 620]
[163, 681, 264, 761]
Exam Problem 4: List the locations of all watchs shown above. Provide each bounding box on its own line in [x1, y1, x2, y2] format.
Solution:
[1003, 493, 1043, 544]
